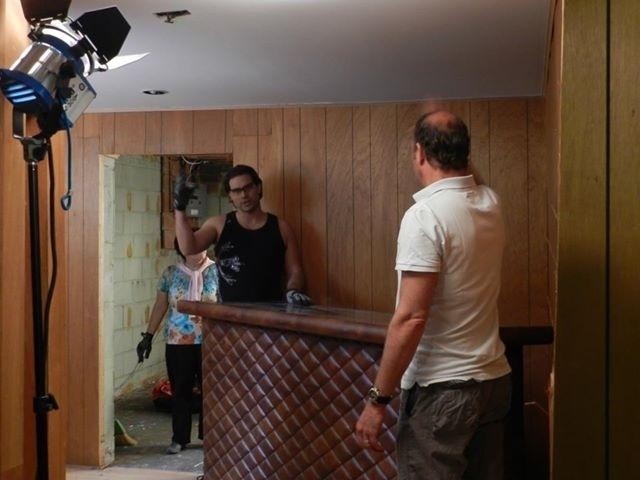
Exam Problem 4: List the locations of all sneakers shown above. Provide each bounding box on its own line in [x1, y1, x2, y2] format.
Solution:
[166, 442, 182, 454]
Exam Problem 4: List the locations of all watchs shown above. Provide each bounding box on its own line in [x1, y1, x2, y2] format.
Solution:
[367, 385, 394, 407]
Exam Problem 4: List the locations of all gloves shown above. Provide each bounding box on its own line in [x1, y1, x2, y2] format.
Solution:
[172, 170, 197, 211]
[285, 288, 313, 307]
[136, 331, 154, 362]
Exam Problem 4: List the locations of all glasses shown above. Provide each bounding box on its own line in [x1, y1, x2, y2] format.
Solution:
[230, 182, 253, 194]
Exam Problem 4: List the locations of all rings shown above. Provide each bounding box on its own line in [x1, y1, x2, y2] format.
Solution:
[362, 439, 370, 444]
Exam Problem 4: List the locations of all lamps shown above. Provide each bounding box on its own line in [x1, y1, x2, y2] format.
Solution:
[1, 0, 134, 480]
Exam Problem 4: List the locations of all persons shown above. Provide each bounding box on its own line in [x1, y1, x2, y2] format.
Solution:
[136, 226, 222, 454]
[173, 163, 314, 305]
[355, 98, 515, 480]
